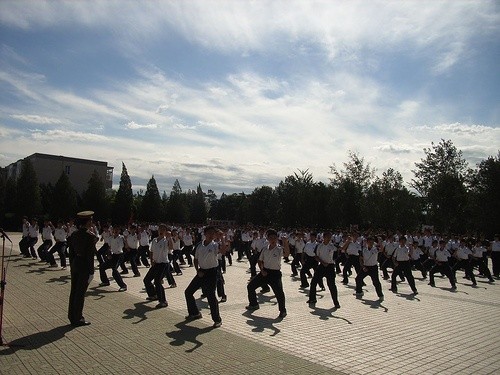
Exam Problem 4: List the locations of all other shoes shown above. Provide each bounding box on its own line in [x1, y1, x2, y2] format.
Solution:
[237, 254, 286, 318]
[19, 253, 67, 270]
[421, 271, 500, 292]
[283, 257, 418, 309]
[96, 253, 232, 327]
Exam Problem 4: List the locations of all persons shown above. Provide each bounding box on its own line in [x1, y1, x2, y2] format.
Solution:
[24, 216, 39, 260]
[140, 224, 174, 307]
[47, 218, 70, 270]
[469, 240, 496, 283]
[98, 223, 128, 293]
[184, 226, 222, 328]
[36, 218, 55, 263]
[65, 210, 96, 325]
[490, 234, 500, 280]
[307, 231, 342, 307]
[452, 239, 477, 286]
[353, 238, 384, 302]
[19, 215, 31, 258]
[426, 238, 457, 292]
[35, 217, 498, 305]
[388, 236, 419, 295]
[244, 230, 289, 318]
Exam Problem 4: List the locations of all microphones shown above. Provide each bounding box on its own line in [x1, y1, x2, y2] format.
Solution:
[0, 228, 12, 243]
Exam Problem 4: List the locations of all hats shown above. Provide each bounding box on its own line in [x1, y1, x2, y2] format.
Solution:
[76, 210, 94, 216]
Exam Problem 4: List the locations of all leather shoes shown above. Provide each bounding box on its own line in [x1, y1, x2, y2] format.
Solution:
[73, 320, 91, 326]
